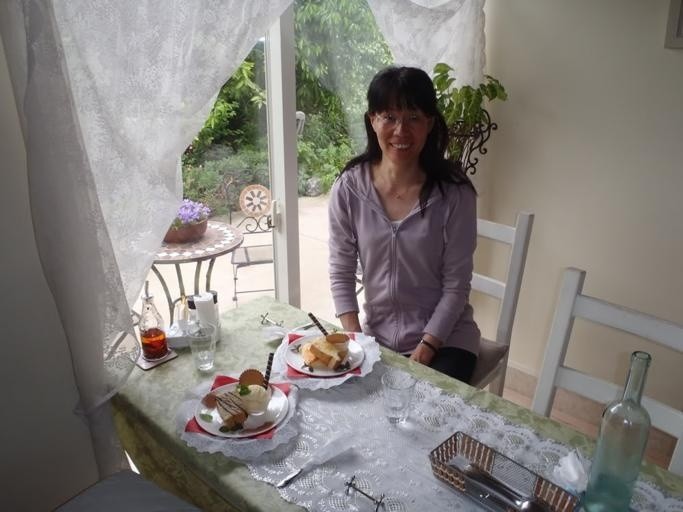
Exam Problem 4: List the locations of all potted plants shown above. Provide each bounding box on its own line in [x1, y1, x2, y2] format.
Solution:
[429, 62, 509, 177]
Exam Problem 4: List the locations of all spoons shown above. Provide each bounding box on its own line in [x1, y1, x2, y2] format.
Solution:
[449, 462, 554, 511]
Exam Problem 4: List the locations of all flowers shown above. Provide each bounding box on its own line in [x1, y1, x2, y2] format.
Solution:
[168, 199, 211, 230]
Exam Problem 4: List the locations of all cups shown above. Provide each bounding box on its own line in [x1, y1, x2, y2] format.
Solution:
[381, 370, 414, 425]
[187, 324, 222, 372]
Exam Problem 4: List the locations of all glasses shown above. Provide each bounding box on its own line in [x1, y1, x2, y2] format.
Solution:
[373, 111, 428, 127]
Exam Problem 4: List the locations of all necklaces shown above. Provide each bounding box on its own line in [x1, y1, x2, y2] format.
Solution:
[377, 164, 421, 199]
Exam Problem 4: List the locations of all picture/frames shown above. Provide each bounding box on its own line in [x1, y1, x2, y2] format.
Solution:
[663, 0, 683, 48]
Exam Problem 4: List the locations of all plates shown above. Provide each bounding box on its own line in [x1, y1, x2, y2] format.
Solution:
[192, 381, 290, 437]
[285, 334, 366, 377]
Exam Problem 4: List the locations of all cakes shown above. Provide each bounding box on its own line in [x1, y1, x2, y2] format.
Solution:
[214, 352, 275, 431]
[299, 312, 352, 372]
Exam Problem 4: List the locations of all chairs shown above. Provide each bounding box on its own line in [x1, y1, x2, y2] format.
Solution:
[103, 280, 142, 363]
[461, 210, 534, 405]
[532, 267, 683, 478]
[53, 471, 205, 512]
[221, 168, 277, 302]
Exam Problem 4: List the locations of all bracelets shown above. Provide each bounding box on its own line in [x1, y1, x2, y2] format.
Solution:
[420, 339, 437, 353]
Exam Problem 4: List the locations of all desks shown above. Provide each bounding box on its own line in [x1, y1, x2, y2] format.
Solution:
[149, 219, 244, 331]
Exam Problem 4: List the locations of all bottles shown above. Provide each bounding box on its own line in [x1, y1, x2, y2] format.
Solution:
[579, 351, 654, 510]
[140, 296, 166, 359]
[186, 289, 221, 331]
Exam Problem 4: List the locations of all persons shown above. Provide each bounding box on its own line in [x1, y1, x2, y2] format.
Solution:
[327, 66, 481, 386]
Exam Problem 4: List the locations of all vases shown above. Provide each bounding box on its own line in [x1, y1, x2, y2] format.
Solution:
[164, 221, 208, 244]
